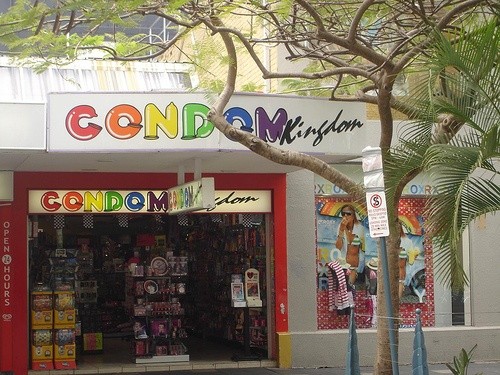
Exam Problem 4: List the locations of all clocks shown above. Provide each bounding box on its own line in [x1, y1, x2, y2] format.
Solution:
[151, 257, 170, 278]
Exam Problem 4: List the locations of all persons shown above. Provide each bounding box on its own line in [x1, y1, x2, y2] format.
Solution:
[398, 220, 407, 299]
[367, 257, 378, 328]
[336, 204, 366, 291]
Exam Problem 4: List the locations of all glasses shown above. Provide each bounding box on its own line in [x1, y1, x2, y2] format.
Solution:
[341, 211, 353, 216]
